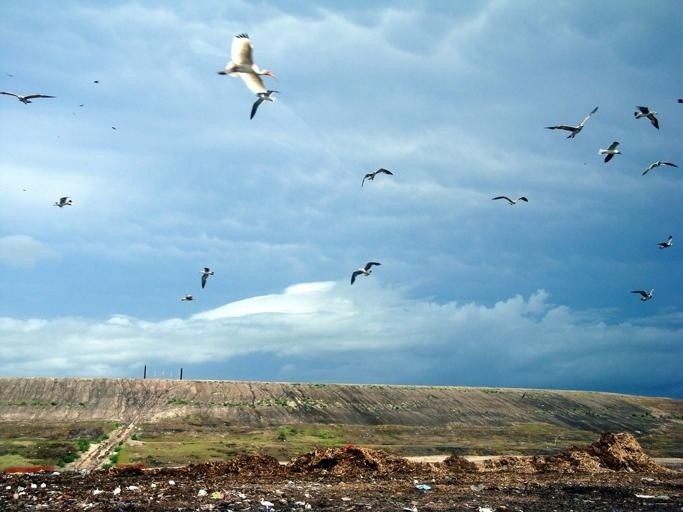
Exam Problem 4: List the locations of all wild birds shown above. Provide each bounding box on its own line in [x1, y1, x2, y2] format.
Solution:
[199, 268, 214, 288]
[544, 103, 678, 175]
[655, 235, 673, 249]
[630, 288, 655, 301]
[250, 90, 280, 119]
[52, 196, 73, 207]
[0, 92, 56, 104]
[351, 262, 382, 285]
[181, 295, 193, 300]
[216, 32, 279, 94]
[362, 168, 393, 187]
[491, 196, 528, 205]
